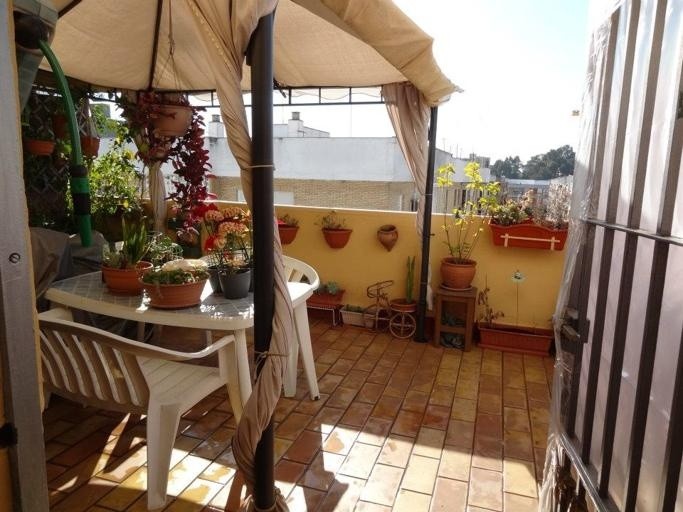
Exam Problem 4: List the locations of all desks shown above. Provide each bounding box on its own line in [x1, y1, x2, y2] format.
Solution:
[44, 267, 320, 429]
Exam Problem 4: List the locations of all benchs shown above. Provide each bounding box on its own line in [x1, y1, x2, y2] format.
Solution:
[308, 289, 345, 327]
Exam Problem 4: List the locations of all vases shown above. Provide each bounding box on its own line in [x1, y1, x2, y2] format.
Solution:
[377, 225, 398, 252]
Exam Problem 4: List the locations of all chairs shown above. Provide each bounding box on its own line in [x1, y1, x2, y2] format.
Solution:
[32, 304, 244, 511]
[195, 250, 321, 404]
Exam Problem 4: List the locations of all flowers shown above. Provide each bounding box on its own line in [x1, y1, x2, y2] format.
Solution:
[161, 259, 208, 272]
[102, 86, 252, 256]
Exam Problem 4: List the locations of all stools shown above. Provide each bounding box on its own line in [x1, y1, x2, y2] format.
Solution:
[434, 287, 477, 352]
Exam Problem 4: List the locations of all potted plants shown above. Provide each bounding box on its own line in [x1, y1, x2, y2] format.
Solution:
[137, 272, 210, 308]
[30, 112, 101, 156]
[207, 264, 234, 294]
[435, 157, 571, 292]
[389, 254, 422, 325]
[340, 304, 375, 328]
[217, 268, 250, 300]
[277, 213, 300, 245]
[98, 203, 155, 297]
[241, 260, 254, 293]
[475, 282, 559, 358]
[315, 210, 352, 248]
